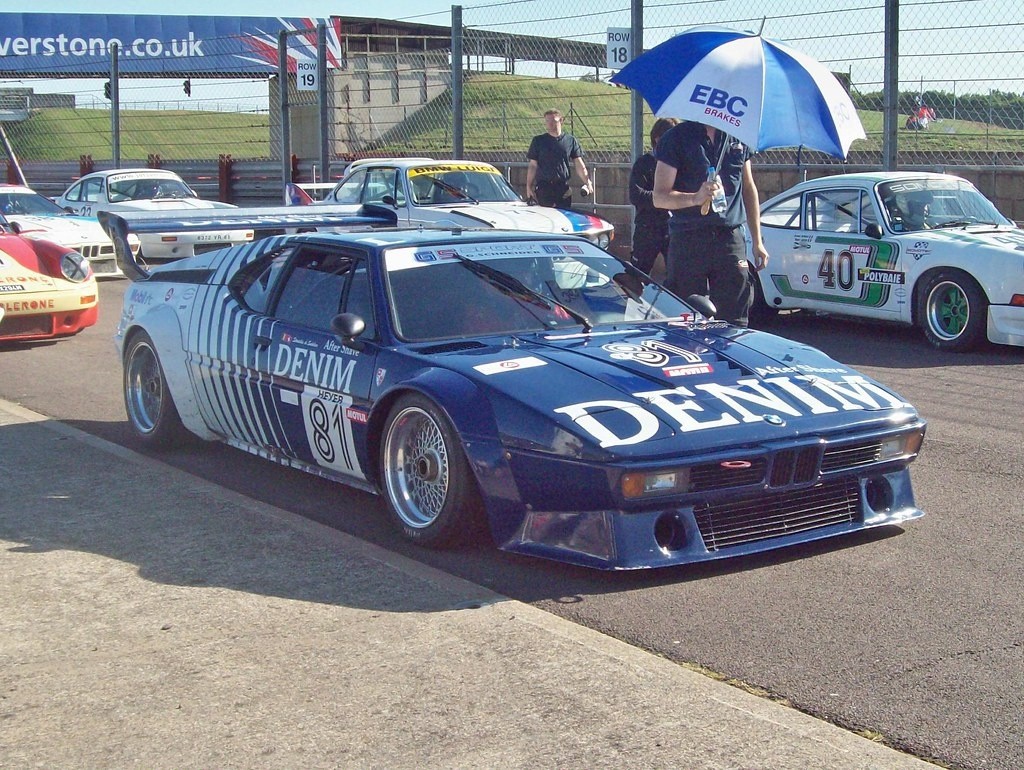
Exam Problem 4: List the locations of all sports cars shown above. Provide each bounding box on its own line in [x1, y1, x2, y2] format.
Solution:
[1, 228, 100, 342]
[284, 156, 616, 261]
[92, 210, 934, 574]
[56, 168, 256, 261]
[744, 172, 1024, 352]
[0, 183, 141, 279]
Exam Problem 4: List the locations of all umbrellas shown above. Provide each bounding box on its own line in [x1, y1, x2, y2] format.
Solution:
[608, 13, 867, 216]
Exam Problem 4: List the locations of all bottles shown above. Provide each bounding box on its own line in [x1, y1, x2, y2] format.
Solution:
[707, 167, 726, 212]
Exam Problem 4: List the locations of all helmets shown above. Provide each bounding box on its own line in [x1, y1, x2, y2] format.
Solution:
[443, 172, 470, 201]
[478, 258, 543, 304]
[895, 190, 933, 222]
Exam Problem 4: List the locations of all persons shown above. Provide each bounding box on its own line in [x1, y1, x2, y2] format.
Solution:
[891, 186, 934, 231]
[132, 177, 177, 201]
[629, 116, 770, 329]
[613, 260, 651, 297]
[443, 172, 488, 201]
[463, 253, 579, 335]
[526, 109, 594, 210]
[904, 107, 937, 131]
[663, 320, 755, 354]
